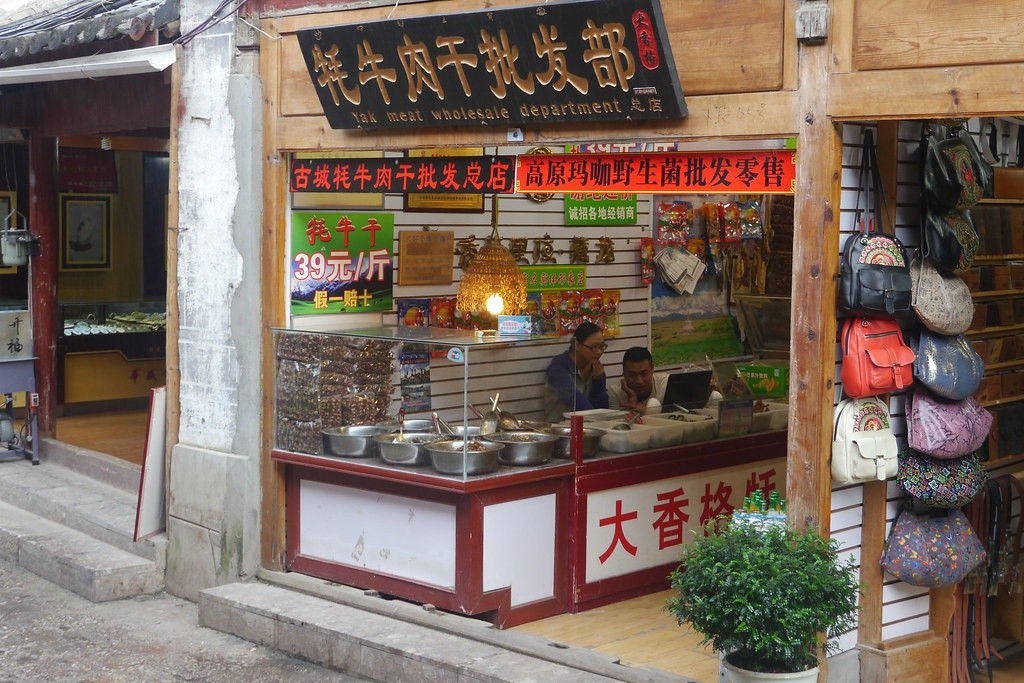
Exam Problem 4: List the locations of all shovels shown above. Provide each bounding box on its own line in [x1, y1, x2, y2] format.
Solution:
[393, 408, 407, 444]
[674, 403, 696, 414]
[488, 398, 520, 430]
[480, 392, 501, 435]
[613, 413, 640, 430]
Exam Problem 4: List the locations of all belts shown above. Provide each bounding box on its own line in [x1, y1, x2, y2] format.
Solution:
[950, 473, 1024, 683]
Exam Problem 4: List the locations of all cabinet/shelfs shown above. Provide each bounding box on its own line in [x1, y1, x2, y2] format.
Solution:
[858, 167, 1024, 683]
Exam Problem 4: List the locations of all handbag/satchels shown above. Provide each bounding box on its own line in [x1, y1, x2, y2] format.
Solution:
[910, 320, 983, 400]
[904, 381, 992, 459]
[921, 120, 987, 209]
[839, 128, 911, 311]
[910, 248, 974, 335]
[922, 201, 978, 274]
[897, 441, 989, 510]
[881, 509, 985, 587]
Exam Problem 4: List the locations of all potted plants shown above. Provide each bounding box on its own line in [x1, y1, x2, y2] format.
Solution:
[659, 514, 868, 683]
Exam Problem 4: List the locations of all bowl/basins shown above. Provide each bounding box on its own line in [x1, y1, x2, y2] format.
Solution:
[321, 425, 388, 457]
[535, 427, 608, 458]
[442, 420, 552, 440]
[423, 440, 505, 476]
[482, 432, 559, 465]
[372, 433, 448, 466]
[376, 419, 434, 433]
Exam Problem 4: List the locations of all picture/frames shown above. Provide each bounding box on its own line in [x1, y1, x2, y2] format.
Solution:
[59, 193, 113, 272]
[0, 191, 18, 273]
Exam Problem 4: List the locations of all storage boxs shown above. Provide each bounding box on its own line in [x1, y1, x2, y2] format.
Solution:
[566, 364, 787, 452]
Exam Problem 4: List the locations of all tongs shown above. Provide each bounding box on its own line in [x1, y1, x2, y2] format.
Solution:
[432, 412, 454, 438]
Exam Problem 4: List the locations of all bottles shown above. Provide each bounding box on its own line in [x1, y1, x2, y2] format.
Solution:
[730, 488, 788, 536]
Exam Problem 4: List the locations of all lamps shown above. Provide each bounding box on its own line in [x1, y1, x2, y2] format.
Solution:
[455, 195, 528, 318]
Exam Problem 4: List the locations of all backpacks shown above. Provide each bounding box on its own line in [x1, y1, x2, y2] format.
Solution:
[840, 314, 915, 399]
[831, 395, 899, 485]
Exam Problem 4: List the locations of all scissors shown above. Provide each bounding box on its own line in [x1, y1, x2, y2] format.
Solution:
[669, 415, 684, 421]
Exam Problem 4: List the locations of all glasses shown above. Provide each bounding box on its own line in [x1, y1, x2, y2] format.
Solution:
[579, 341, 608, 352]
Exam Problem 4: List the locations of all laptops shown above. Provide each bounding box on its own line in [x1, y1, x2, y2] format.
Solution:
[661, 370, 712, 410]
